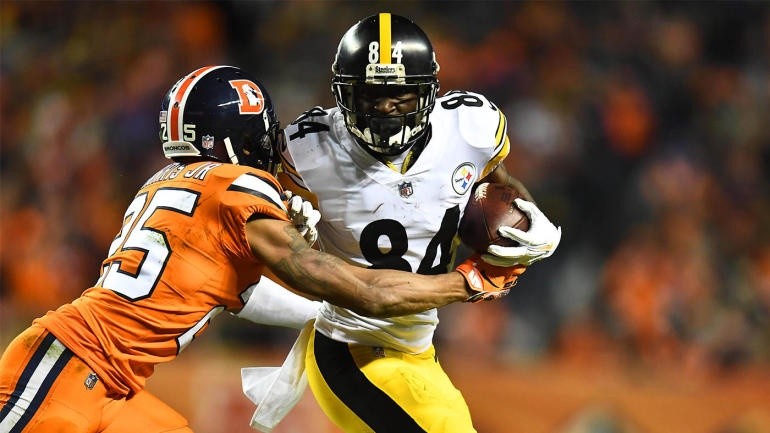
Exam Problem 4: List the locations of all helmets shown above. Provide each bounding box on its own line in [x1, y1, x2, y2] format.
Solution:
[331, 13, 440, 156]
[158, 65, 281, 177]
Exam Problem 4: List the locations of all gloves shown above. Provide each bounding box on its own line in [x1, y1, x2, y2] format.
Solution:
[284, 189, 321, 248]
[480, 198, 562, 266]
[455, 253, 527, 304]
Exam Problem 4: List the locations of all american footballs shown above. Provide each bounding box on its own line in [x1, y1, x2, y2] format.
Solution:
[458, 182, 532, 256]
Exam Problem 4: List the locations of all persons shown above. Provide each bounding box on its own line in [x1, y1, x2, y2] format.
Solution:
[0, 65, 524, 433]
[0, 0, 770, 433]
[278, 14, 561, 433]
